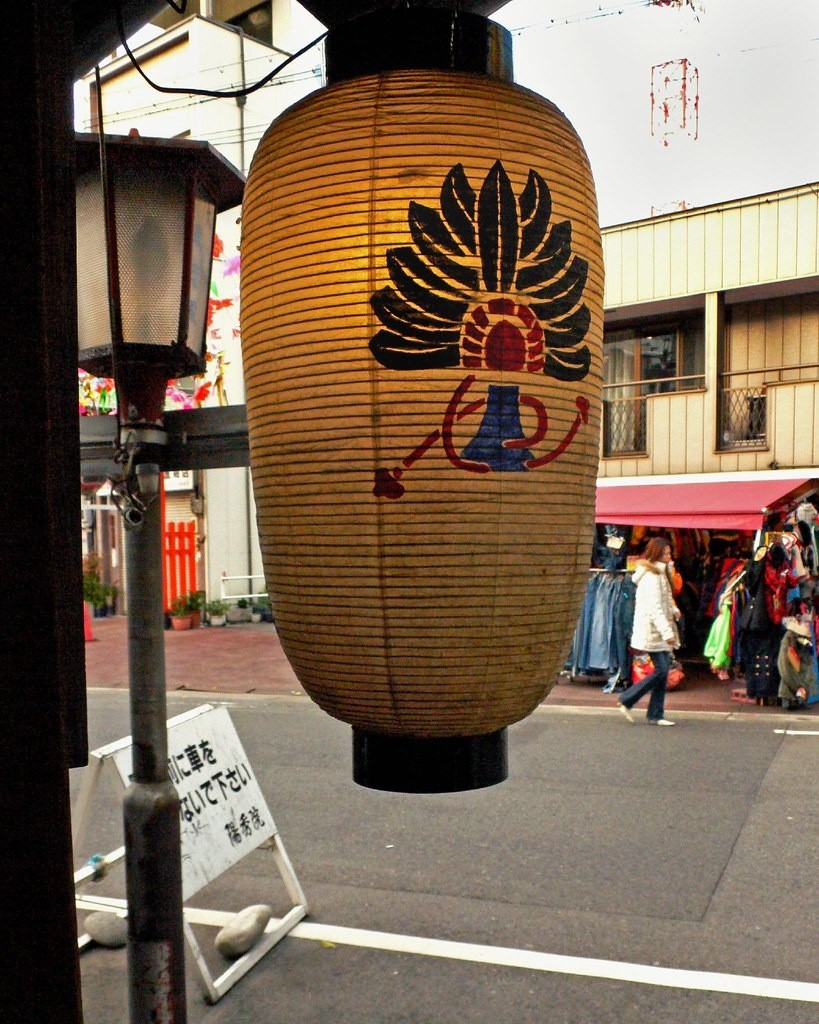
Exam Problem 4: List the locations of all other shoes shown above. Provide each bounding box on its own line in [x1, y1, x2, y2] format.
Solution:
[649, 717, 676, 725]
[619, 704, 634, 723]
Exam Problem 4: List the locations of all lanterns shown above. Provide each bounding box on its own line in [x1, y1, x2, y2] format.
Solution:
[242, 6, 611, 798]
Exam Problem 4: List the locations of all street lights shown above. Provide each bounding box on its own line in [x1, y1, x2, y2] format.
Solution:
[69, 130, 246, 1024]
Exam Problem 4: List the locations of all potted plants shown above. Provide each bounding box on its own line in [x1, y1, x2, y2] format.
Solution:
[165, 587, 274, 631]
[83, 572, 117, 619]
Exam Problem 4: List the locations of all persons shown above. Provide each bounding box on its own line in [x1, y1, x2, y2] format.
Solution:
[616, 537, 680, 726]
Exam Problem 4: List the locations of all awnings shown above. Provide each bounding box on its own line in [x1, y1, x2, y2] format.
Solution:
[588, 470, 814, 531]
